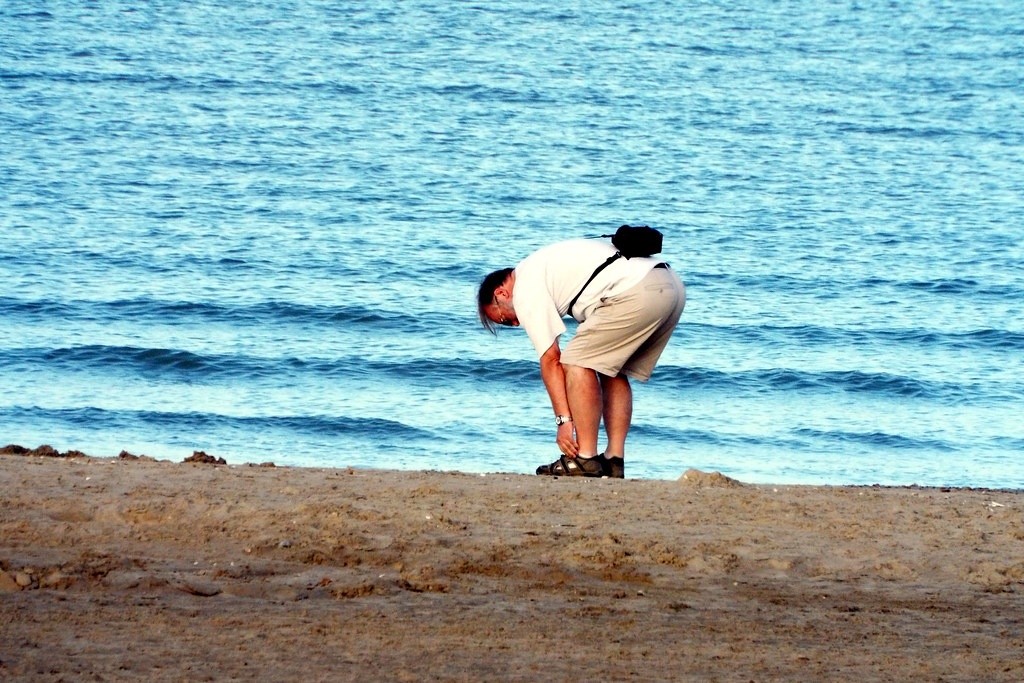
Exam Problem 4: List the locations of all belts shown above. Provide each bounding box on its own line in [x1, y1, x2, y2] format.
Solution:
[654, 262, 670, 268]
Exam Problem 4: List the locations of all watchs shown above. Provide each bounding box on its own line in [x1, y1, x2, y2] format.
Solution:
[555, 416, 573, 426]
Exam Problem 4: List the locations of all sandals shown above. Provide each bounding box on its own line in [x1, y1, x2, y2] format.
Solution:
[536, 454, 604, 477]
[600, 453, 625, 478]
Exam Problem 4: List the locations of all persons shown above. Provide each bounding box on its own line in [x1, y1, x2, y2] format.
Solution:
[476, 238, 687, 478]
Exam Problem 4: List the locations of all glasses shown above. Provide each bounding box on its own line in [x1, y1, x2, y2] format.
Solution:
[495, 296, 512, 327]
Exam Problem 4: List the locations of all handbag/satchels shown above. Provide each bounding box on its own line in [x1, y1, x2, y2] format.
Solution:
[613, 225, 664, 260]
